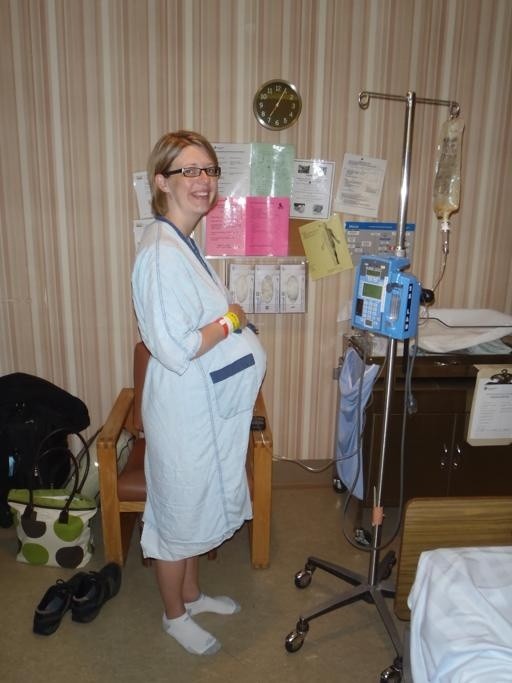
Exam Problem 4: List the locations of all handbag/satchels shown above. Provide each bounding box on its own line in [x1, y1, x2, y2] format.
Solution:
[7, 427, 98, 569]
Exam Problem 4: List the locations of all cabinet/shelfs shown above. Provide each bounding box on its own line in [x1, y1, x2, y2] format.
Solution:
[343, 331, 512, 507]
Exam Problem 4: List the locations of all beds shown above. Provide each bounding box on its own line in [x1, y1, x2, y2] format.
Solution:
[392, 496, 512, 682]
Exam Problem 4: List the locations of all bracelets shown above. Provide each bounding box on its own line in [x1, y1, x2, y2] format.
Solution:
[214, 311, 240, 339]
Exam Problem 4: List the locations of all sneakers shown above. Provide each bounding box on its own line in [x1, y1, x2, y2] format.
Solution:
[32, 562, 122, 635]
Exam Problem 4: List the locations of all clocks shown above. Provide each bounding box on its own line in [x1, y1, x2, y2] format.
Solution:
[251, 76, 306, 131]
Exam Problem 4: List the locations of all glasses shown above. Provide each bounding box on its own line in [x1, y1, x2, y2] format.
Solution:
[162, 166, 221, 177]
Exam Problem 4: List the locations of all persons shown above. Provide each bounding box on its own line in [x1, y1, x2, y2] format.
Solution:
[131, 130, 267, 656]
[321, 223, 340, 266]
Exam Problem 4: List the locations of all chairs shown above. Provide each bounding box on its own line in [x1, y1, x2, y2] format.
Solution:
[98, 331, 275, 571]
